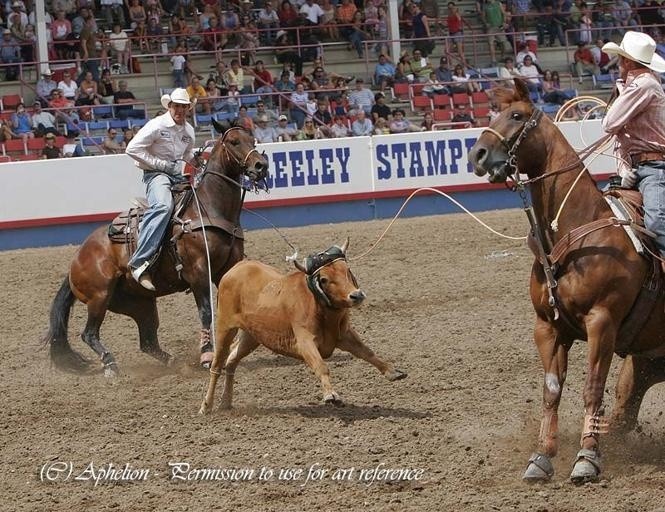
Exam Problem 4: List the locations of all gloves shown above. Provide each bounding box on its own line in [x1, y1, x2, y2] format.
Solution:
[164, 160, 180, 176]
[619, 164, 640, 189]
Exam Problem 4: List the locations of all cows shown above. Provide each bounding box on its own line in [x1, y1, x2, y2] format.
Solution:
[199, 236, 408, 416]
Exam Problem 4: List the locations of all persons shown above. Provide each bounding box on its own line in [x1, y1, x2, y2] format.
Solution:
[123, 87, 197, 292]
[599, 30, 665, 275]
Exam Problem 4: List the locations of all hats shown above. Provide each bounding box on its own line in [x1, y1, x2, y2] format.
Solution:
[40, 69, 55, 76]
[2, 29, 13, 34]
[274, 30, 287, 39]
[160, 88, 198, 111]
[601, 30, 665, 73]
[257, 115, 271, 123]
[32, 100, 41, 106]
[43, 133, 56, 144]
[278, 114, 288, 121]
[392, 107, 406, 117]
[10, 2, 23, 8]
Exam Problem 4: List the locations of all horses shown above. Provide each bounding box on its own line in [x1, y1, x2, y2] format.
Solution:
[468, 77, 664, 485]
[44, 115, 270, 384]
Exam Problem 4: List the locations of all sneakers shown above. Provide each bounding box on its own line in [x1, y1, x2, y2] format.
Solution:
[129, 267, 155, 290]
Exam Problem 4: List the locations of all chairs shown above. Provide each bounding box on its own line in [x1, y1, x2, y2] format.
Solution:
[0, 1, 665, 162]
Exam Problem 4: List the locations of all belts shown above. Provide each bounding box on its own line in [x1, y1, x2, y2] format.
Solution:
[632, 149, 665, 163]
